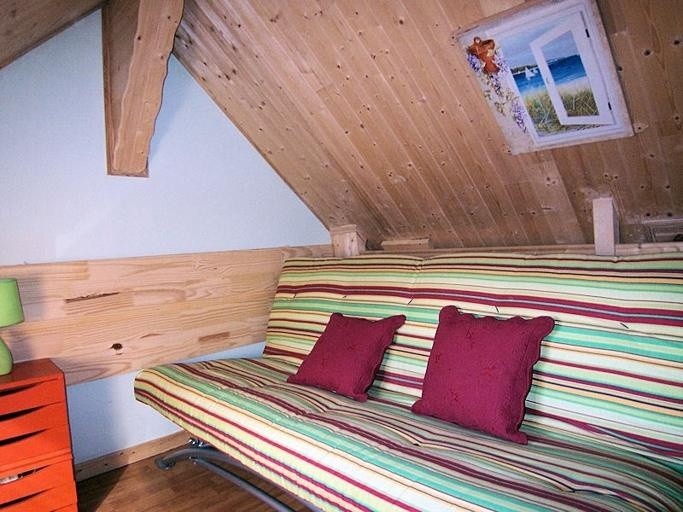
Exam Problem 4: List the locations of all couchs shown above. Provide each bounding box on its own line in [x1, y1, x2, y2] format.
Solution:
[133, 253, 683, 511]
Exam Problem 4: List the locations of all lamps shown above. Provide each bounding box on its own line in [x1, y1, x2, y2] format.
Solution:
[0, 277, 24, 376]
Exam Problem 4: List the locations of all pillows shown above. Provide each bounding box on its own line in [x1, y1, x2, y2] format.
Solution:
[411, 305, 554, 445]
[286, 312, 406, 403]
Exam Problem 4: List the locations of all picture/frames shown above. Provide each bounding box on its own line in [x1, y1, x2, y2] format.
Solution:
[452, 0, 633, 157]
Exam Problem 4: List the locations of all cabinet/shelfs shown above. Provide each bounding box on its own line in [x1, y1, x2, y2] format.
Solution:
[0, 357, 78, 511]
